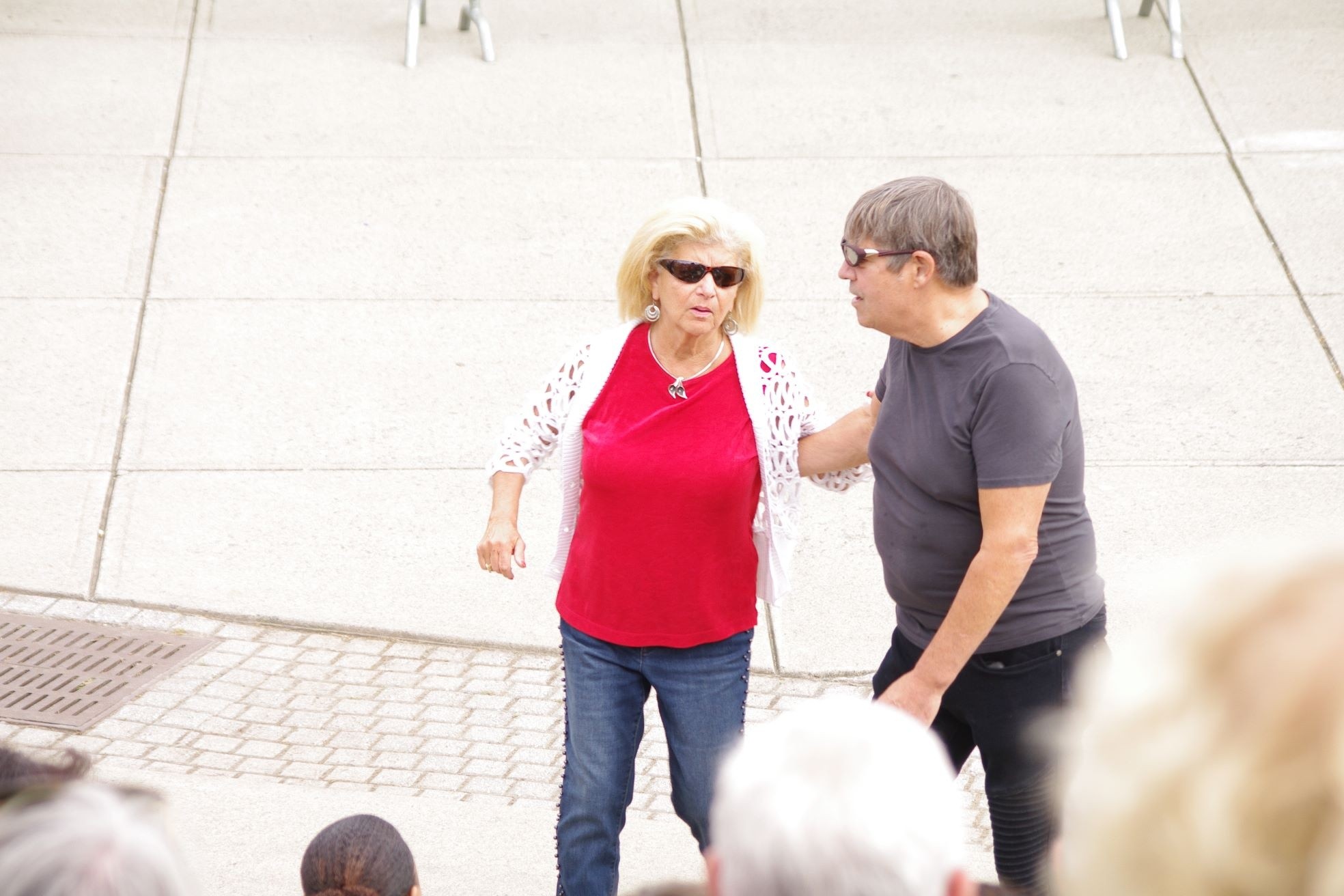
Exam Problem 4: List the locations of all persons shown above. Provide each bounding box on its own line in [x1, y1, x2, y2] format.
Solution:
[795, 176, 1108, 895]
[477, 200, 872, 895]
[0, 559, 1344, 896]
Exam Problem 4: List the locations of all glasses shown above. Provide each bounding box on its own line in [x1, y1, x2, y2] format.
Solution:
[660, 260, 747, 288]
[840, 240, 917, 266]
